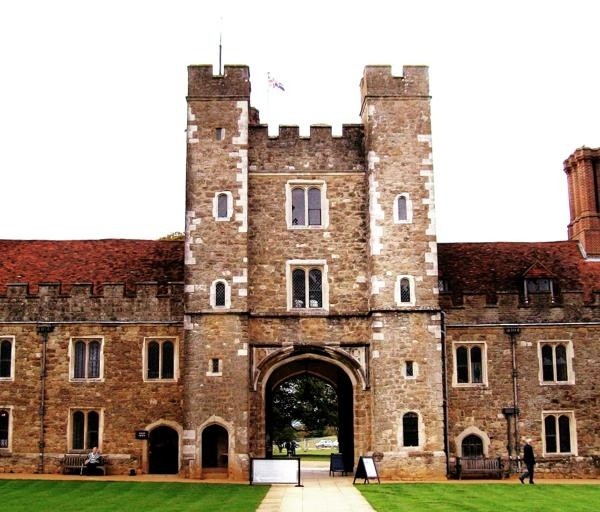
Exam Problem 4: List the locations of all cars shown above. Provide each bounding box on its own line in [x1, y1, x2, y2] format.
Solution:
[290, 440, 300, 448]
[315, 440, 339, 449]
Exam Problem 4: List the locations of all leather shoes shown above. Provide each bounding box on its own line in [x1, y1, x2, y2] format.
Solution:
[529, 481, 534, 484]
[519, 477, 524, 484]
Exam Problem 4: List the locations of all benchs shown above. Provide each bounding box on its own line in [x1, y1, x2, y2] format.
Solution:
[453, 454, 505, 480]
[61, 452, 111, 475]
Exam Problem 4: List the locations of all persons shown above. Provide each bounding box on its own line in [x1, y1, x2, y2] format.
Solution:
[519, 438, 536, 486]
[84, 446, 101, 476]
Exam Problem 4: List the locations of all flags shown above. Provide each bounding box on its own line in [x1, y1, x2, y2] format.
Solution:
[267, 74, 284, 91]
